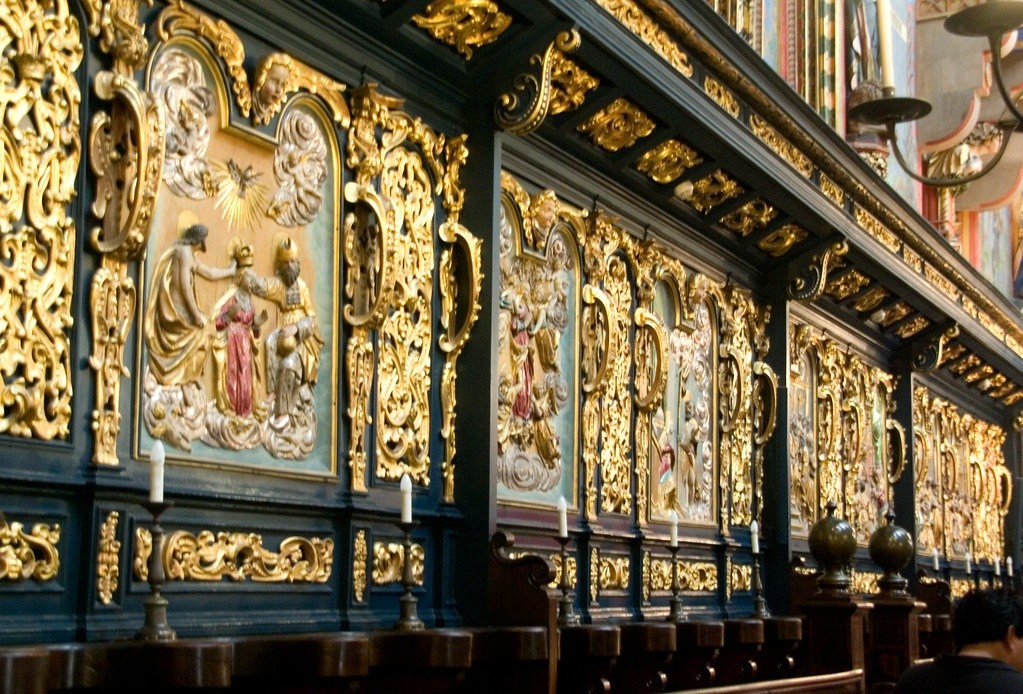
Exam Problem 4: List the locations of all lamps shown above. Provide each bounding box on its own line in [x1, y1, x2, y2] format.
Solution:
[847, 0, 1023, 187]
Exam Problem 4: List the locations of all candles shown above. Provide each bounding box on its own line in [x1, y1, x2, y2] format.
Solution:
[557, 495, 568, 537]
[400, 473, 413, 522]
[750, 520, 760, 552]
[994, 556, 1000, 575]
[965, 553, 971, 573]
[1006, 557, 1013, 575]
[149, 439, 166, 503]
[932, 548, 939, 569]
[669, 512, 679, 546]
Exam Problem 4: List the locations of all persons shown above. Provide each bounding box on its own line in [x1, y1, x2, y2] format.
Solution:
[145, 224, 237, 384]
[896, 587, 1023, 694]
[216, 241, 268, 413]
[255, 238, 315, 417]
